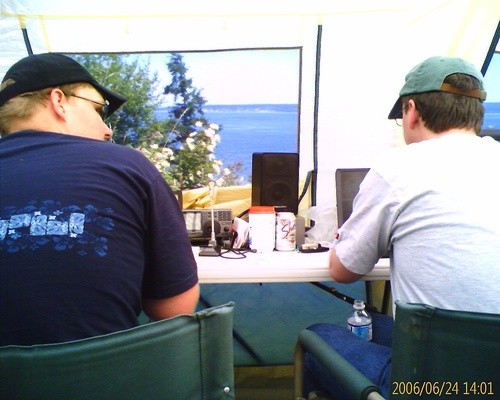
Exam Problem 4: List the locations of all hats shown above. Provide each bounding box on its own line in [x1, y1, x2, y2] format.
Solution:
[0, 53, 125, 118]
[388, 56, 486, 120]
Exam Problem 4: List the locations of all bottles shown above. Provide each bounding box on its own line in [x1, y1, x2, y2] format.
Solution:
[346, 299, 374, 343]
[248, 206, 276, 253]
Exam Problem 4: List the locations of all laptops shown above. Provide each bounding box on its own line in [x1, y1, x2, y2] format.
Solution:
[334, 168, 391, 258]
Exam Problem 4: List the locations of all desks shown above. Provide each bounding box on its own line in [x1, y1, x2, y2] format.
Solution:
[195, 250, 393, 363]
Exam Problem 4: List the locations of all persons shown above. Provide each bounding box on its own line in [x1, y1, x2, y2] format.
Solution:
[301, 56, 500, 400]
[0, 52, 199, 348]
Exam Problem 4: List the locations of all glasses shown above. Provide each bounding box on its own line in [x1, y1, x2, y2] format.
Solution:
[47, 88, 111, 116]
[394, 98, 415, 126]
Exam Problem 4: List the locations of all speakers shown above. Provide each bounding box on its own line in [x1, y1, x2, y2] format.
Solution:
[251, 152, 299, 215]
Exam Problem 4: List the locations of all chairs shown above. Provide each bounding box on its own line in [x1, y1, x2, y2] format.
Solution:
[0, 301, 235, 400]
[293, 300, 500, 400]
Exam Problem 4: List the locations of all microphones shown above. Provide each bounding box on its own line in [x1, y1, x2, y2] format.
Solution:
[199, 181, 221, 257]
